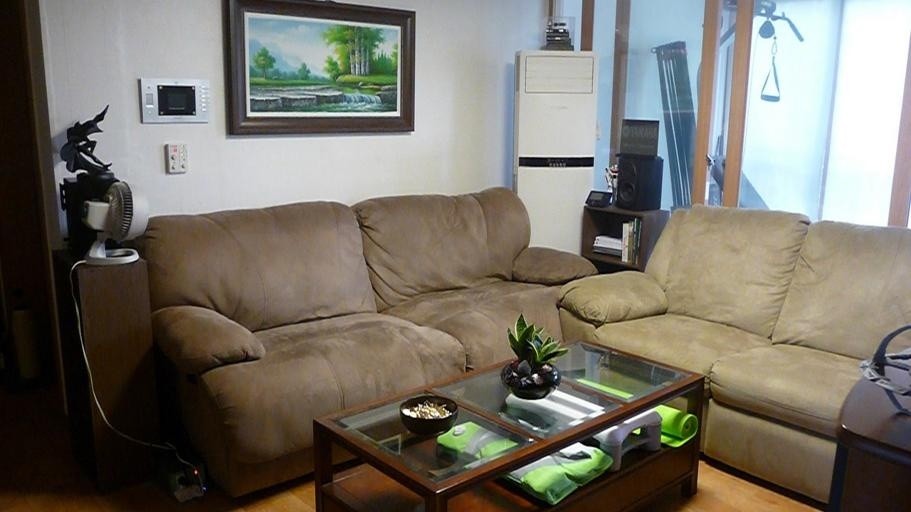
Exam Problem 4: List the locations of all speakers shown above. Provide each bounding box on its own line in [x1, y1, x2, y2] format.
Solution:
[616, 156, 664, 210]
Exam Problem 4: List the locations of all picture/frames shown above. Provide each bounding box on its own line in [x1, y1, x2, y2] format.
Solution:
[219, 1, 418, 136]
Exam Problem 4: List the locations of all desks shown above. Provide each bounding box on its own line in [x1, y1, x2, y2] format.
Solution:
[826, 355, 911, 509]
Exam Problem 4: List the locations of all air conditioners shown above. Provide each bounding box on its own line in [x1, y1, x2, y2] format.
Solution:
[512, 50, 598, 258]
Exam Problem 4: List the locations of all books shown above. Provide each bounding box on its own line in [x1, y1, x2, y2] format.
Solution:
[592, 216, 644, 267]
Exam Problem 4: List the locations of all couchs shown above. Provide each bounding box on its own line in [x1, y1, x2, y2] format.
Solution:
[142, 187, 601, 503]
[551, 201, 910, 510]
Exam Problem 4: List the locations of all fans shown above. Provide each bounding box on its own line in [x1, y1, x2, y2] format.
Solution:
[79, 179, 144, 268]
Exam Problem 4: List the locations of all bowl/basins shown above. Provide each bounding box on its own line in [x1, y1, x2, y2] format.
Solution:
[399, 395, 459, 438]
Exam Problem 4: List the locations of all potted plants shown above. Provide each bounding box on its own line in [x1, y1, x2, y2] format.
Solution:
[503, 315, 568, 399]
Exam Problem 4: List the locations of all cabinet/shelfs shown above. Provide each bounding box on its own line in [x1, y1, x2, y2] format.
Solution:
[56, 250, 164, 494]
[580, 205, 669, 276]
